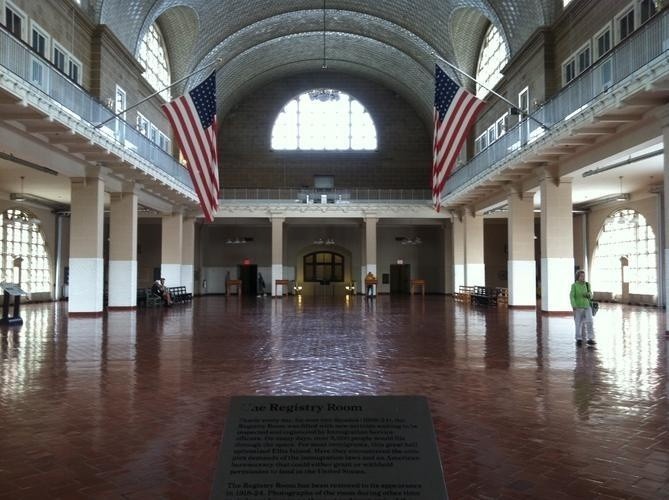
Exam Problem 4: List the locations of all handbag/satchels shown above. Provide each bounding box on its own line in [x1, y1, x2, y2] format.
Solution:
[591, 302, 599, 316]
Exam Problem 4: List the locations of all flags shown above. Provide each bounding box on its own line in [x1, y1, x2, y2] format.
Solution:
[161, 69, 221, 223]
[432, 63, 487, 214]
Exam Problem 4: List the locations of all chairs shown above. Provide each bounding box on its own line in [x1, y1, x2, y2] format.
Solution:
[138, 286, 192, 309]
[452, 285, 507, 306]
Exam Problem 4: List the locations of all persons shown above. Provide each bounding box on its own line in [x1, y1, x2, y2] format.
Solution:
[570, 269, 597, 345]
[368, 272, 373, 297]
[256, 273, 267, 298]
[151, 278, 174, 307]
[225, 272, 230, 296]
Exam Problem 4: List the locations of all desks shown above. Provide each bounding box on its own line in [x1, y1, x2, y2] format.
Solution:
[226, 280, 241, 296]
[275, 280, 289, 297]
[411, 279, 425, 295]
[365, 280, 377, 297]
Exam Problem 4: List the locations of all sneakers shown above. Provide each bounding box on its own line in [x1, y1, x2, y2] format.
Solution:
[576, 340, 582, 345]
[587, 340, 596, 345]
